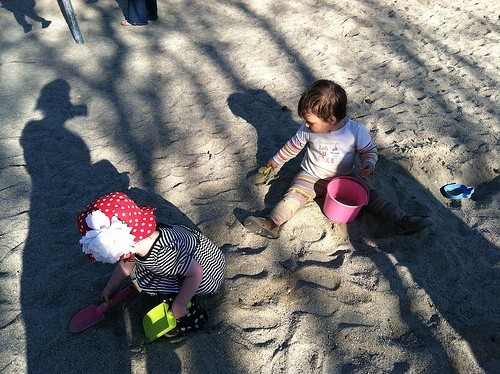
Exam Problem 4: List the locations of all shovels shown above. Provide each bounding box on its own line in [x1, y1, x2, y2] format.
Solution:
[142, 300, 193, 344]
[67, 285, 133, 334]
[250, 165, 274, 185]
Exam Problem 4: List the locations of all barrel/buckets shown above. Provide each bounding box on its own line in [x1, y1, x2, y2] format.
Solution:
[324, 176, 369, 224]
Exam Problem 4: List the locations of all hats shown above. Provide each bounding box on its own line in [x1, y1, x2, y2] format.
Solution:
[75, 190, 158, 265]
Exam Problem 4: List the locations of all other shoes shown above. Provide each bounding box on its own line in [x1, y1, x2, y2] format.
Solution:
[120, 19, 134, 26]
[243, 215, 280, 239]
[162, 307, 207, 339]
[394, 214, 435, 236]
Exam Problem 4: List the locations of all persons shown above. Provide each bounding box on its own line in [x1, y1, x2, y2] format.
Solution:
[76, 191, 226, 338]
[244, 79, 433, 239]
[115, 0, 158, 26]
